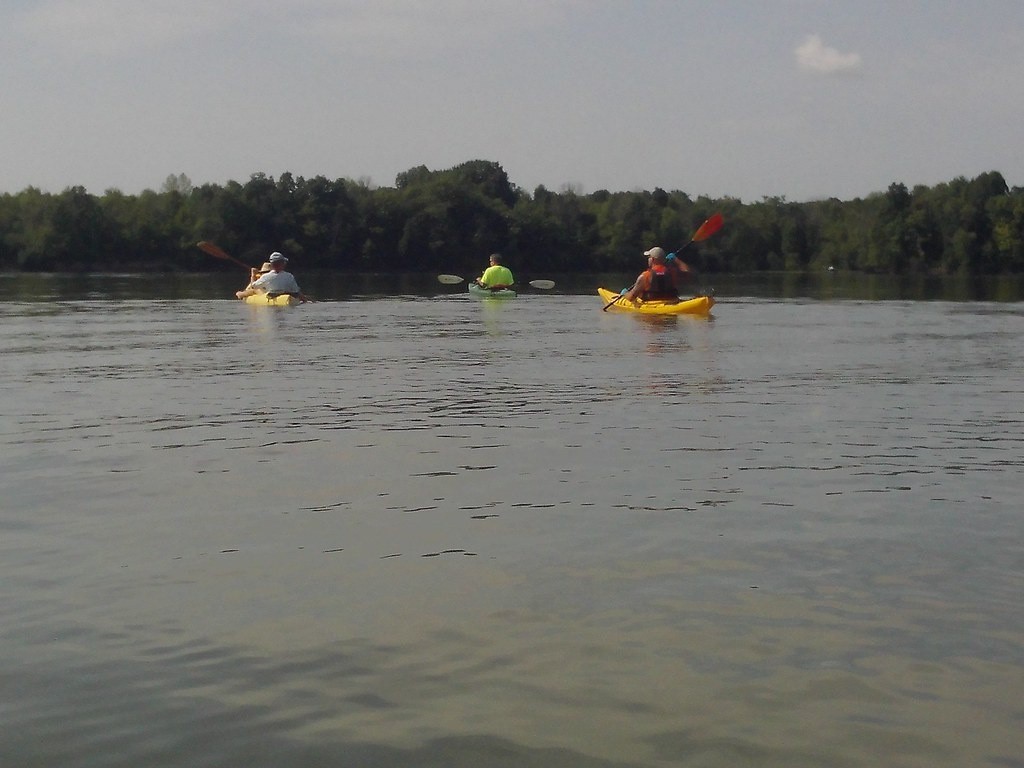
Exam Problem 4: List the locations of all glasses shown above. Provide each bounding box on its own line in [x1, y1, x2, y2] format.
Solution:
[489, 260, 492, 263]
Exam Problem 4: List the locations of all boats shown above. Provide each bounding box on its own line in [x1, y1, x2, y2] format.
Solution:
[241, 275, 292, 306]
[466, 282, 517, 300]
[594, 285, 718, 316]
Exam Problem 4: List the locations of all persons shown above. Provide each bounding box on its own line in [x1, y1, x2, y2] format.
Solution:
[620, 247, 689, 303]
[475, 253, 515, 290]
[236, 252, 301, 299]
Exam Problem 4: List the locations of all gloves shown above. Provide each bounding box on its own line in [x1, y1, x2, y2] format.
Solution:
[620, 287, 628, 295]
[666, 252, 677, 263]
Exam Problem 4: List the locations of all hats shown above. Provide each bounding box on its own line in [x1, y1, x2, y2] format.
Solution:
[258, 262, 271, 272]
[643, 247, 665, 260]
[270, 252, 288, 263]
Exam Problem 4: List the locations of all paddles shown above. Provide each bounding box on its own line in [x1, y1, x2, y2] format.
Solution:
[602, 213, 724, 311]
[196, 240, 252, 269]
[437, 274, 556, 290]
